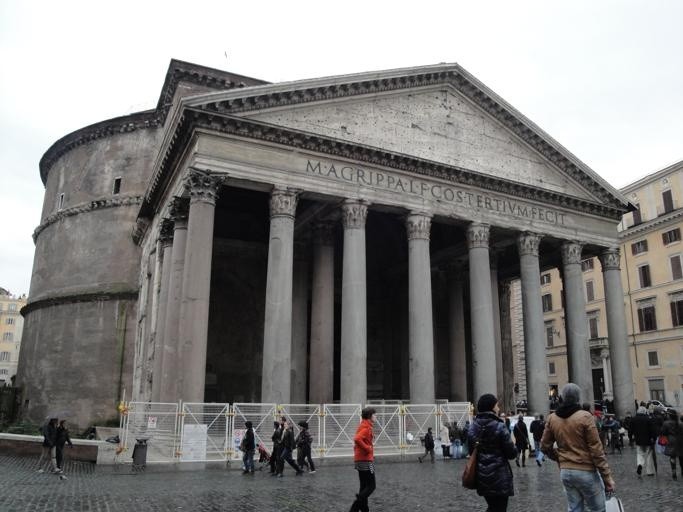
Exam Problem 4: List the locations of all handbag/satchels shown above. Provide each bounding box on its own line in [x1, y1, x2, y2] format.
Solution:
[658, 434, 670, 446]
[605, 491, 624, 512]
[462, 448, 477, 488]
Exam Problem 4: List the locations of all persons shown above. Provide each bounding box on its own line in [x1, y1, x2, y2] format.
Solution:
[56, 420, 73, 468]
[418, 383, 683, 512]
[34, 418, 61, 473]
[349, 406, 377, 512]
[240, 416, 316, 478]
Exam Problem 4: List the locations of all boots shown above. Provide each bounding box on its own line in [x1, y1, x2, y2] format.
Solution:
[516, 457, 520, 467]
[522, 456, 526, 466]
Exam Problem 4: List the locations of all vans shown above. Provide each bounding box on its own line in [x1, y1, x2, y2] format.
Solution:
[648, 400, 673, 415]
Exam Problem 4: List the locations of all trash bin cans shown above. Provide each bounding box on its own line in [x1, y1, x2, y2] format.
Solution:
[133, 438, 148, 468]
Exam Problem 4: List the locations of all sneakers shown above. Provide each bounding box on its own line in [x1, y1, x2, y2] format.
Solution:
[637, 465, 642, 475]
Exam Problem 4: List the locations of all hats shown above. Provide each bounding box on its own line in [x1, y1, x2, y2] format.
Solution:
[665, 409, 676, 416]
[477, 394, 497, 411]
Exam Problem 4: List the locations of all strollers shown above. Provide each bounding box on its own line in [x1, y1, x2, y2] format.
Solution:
[255, 443, 271, 471]
